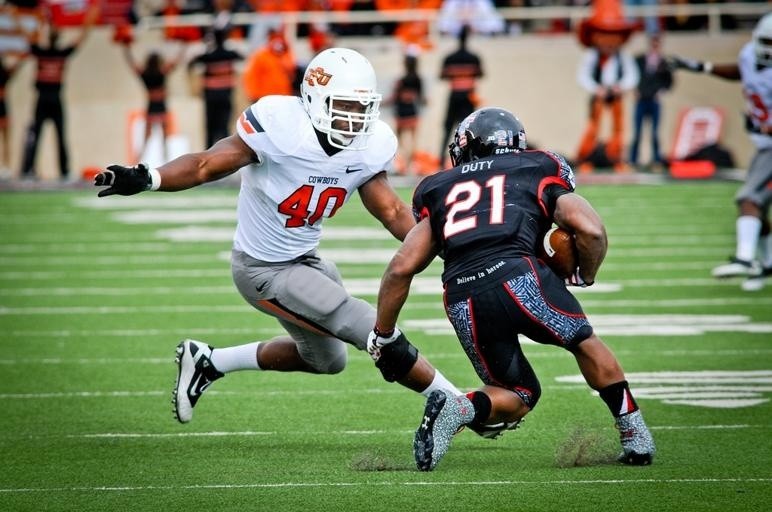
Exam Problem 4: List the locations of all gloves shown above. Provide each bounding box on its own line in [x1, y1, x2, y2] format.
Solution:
[93, 162, 162, 198]
[672, 54, 704, 72]
[366, 324, 402, 363]
[564, 266, 595, 289]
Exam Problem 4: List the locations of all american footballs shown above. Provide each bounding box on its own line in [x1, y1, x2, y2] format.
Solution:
[536, 228, 577, 280]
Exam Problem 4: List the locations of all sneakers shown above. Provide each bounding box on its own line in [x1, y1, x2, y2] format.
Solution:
[710, 254, 772, 293]
[413, 389, 476, 472]
[466, 417, 525, 441]
[612, 408, 656, 466]
[171, 339, 225, 424]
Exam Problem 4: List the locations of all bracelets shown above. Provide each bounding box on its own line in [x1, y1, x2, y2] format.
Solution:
[149, 169, 161, 190]
[703, 61, 713, 73]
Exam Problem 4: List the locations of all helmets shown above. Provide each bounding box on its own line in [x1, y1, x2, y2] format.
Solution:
[448, 107, 529, 167]
[752, 12, 772, 67]
[300, 47, 384, 151]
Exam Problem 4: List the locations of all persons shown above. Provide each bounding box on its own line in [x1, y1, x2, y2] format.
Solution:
[95, 49, 524, 438]
[671, 13, 772, 292]
[366, 107, 656, 471]
